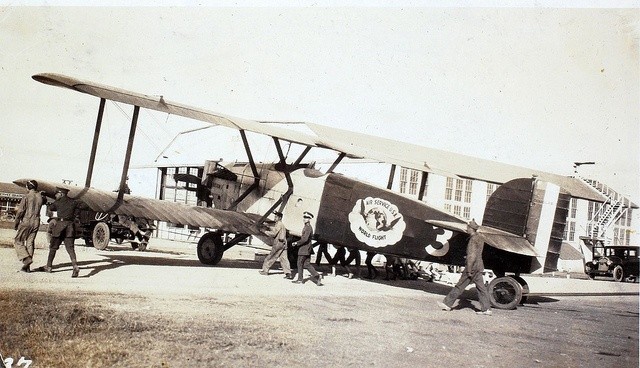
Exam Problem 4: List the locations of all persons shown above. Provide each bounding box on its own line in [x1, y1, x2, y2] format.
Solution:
[14, 181, 42, 272]
[39, 186, 80, 278]
[436, 218, 493, 315]
[362, 251, 380, 279]
[292, 212, 322, 286]
[327, 244, 354, 279]
[312, 240, 332, 271]
[382, 254, 399, 281]
[258, 212, 293, 279]
[342, 247, 362, 280]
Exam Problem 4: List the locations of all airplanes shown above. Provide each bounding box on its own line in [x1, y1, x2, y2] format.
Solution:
[11, 72, 633, 325]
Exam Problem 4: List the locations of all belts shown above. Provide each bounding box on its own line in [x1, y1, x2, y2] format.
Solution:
[57, 218, 73, 222]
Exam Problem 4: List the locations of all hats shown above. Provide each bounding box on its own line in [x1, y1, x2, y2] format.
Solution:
[27, 180, 39, 192]
[55, 186, 71, 195]
[468, 222, 480, 231]
[303, 212, 315, 220]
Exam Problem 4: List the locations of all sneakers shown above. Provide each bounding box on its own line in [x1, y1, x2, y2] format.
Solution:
[437, 301, 452, 312]
[292, 280, 305, 285]
[316, 275, 323, 287]
[477, 311, 493, 317]
[39, 265, 53, 274]
[72, 267, 80, 278]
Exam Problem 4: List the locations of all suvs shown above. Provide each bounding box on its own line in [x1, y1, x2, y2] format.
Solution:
[585, 246, 640, 282]
[47, 208, 153, 251]
